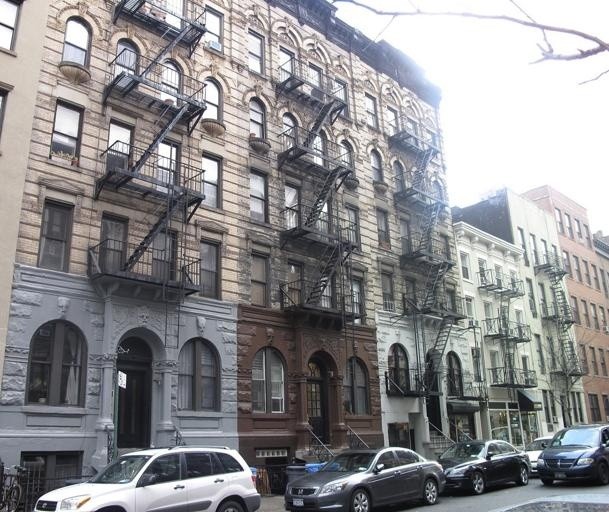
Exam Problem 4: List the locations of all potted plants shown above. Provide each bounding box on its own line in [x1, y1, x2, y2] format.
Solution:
[50, 151, 78, 165]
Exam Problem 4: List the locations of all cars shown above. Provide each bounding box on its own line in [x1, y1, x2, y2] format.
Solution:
[32, 445, 260, 512]
[284, 446, 448, 512]
[521, 424, 609, 485]
[437, 439, 532, 495]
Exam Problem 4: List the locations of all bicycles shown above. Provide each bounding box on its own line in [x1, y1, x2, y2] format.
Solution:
[0, 465, 28, 512]
